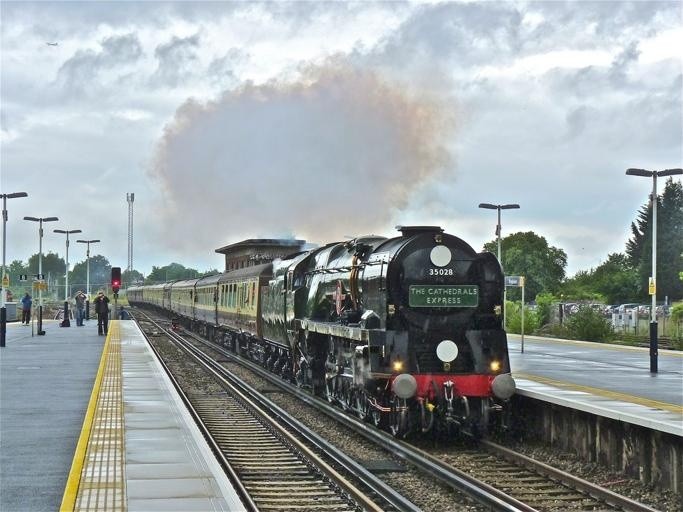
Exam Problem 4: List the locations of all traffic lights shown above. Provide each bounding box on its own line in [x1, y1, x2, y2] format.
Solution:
[111, 267, 121, 294]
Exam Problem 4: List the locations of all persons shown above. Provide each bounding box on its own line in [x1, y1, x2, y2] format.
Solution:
[92, 290, 110, 335]
[74, 290, 86, 326]
[19, 291, 31, 326]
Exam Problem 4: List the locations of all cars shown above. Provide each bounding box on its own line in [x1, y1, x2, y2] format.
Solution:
[585, 301, 669, 319]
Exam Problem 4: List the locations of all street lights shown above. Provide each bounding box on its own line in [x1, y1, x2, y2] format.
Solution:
[53, 229, 82, 327]
[625, 167, 683, 374]
[76, 239, 101, 321]
[0, 191, 28, 347]
[23, 216, 59, 336]
[478, 202, 520, 264]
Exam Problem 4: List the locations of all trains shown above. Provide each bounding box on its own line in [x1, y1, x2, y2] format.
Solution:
[126, 225, 516, 441]
